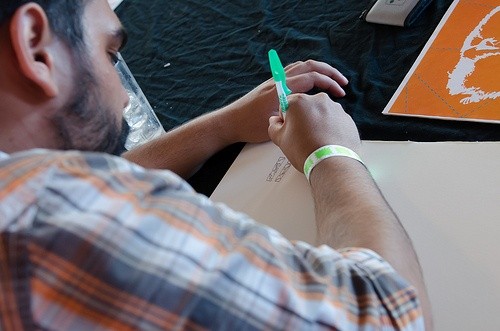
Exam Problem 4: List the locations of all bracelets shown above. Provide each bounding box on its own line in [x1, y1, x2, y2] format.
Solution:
[303, 144, 369, 183]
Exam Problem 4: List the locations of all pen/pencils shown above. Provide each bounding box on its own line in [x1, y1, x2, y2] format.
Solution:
[268, 49, 292, 122]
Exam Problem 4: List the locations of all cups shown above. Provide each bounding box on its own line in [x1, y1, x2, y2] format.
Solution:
[113, 53, 166, 153]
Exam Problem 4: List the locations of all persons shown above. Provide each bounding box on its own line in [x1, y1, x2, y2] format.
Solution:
[0, 0, 434, 331]
